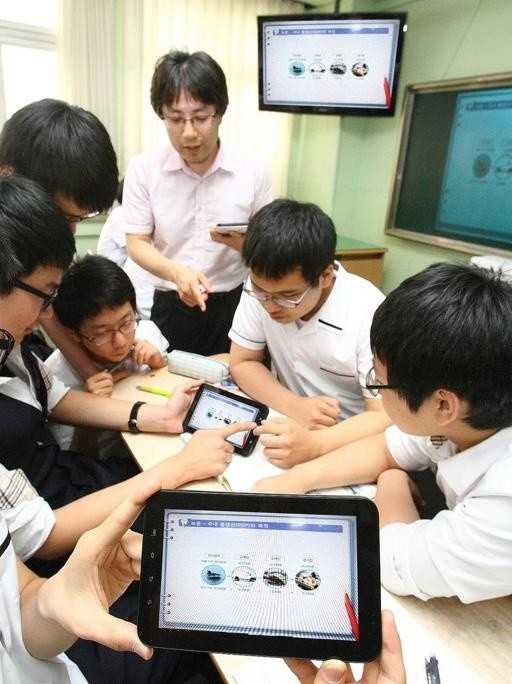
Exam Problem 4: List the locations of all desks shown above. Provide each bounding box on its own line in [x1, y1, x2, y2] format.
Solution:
[335, 234, 389, 289]
[110, 364, 512, 680]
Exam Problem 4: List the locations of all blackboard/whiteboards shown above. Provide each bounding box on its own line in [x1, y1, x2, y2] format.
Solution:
[384, 70, 512, 262]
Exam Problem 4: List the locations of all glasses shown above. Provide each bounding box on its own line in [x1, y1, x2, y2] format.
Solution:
[79, 308, 141, 345]
[243, 269, 312, 308]
[159, 111, 216, 128]
[1, 329, 15, 365]
[65, 211, 98, 223]
[366, 367, 394, 397]
[15, 279, 59, 306]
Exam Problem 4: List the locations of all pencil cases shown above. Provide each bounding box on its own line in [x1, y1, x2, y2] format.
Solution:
[167, 349, 230, 385]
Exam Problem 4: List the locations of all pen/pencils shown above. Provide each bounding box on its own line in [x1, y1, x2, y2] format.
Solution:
[137, 385, 172, 397]
[344, 590, 359, 642]
[242, 432, 247, 447]
[384, 77, 390, 107]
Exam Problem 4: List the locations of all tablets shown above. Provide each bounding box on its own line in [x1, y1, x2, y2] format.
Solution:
[215, 222, 248, 234]
[137, 489, 382, 662]
[182, 383, 269, 457]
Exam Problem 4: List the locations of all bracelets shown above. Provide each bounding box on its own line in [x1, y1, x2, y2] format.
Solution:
[129, 401, 146, 434]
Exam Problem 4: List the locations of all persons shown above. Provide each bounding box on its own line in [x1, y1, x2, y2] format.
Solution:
[0, 99, 131, 385]
[250, 262, 512, 605]
[122, 49, 274, 372]
[0, 477, 407, 684]
[96, 178, 128, 268]
[227, 199, 394, 470]
[0, 174, 257, 564]
[43, 255, 170, 459]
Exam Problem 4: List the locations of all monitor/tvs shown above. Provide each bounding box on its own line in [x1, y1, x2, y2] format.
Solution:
[257, 12, 407, 117]
